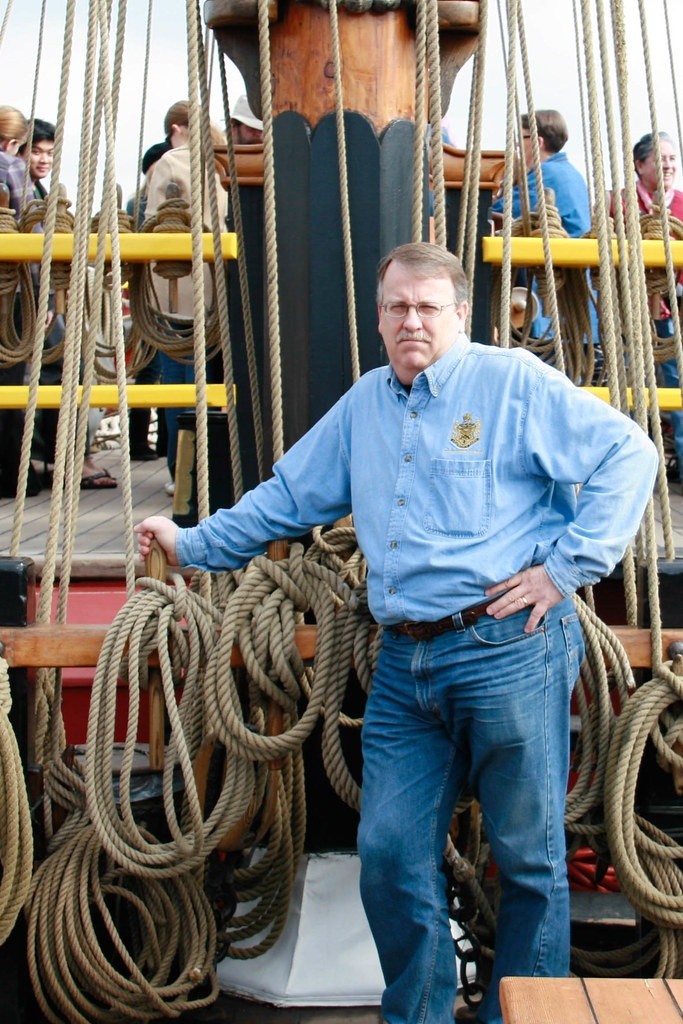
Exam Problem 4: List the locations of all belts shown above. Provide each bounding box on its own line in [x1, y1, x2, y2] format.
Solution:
[381, 588, 506, 638]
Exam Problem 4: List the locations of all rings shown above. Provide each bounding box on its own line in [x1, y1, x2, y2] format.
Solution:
[522, 596, 528, 607]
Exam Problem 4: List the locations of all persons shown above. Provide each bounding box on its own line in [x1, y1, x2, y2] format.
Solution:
[127, 101, 228, 497]
[134, 242, 659, 1024]
[223, 95, 263, 143]
[593, 132, 683, 483]
[490, 110, 606, 386]
[0, 104, 119, 498]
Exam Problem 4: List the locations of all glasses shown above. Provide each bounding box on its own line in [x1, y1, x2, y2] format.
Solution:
[379, 300, 457, 318]
[523, 134, 532, 137]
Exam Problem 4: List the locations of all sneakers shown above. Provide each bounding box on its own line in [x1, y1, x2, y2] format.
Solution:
[131, 448, 158, 460]
[164, 480, 176, 495]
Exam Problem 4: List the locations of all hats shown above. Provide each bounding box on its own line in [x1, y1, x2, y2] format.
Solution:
[221, 94, 263, 133]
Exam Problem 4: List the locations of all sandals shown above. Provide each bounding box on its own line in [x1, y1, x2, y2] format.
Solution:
[82, 467, 119, 489]
